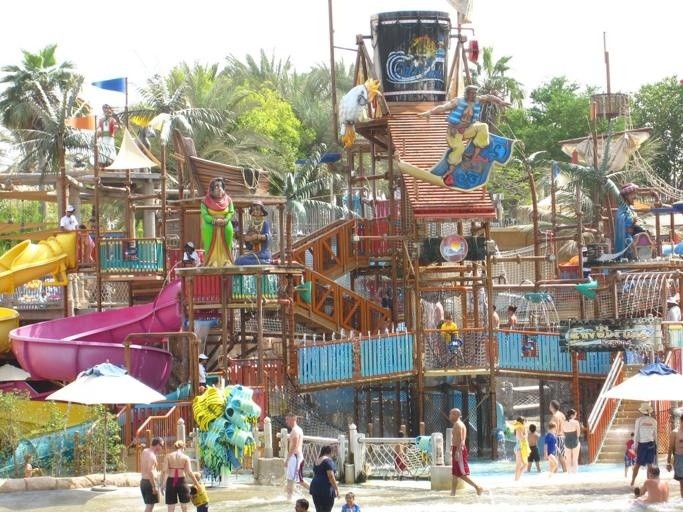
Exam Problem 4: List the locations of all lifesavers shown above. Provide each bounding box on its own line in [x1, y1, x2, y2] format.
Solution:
[440, 234, 468, 263]
[416, 434, 433, 452]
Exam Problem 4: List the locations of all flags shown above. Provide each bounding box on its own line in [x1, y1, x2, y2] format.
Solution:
[91, 77, 126, 94]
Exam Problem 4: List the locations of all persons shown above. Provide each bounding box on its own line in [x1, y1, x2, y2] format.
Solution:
[190, 471, 209, 512]
[58, 204, 95, 266]
[630, 465, 669, 512]
[394, 444, 419, 480]
[200, 176, 272, 267]
[415, 85, 512, 179]
[624, 432, 635, 478]
[374, 280, 519, 370]
[612, 183, 639, 261]
[665, 296, 681, 321]
[23, 454, 36, 477]
[160, 440, 202, 512]
[139, 436, 165, 512]
[630, 402, 658, 487]
[96, 104, 119, 166]
[449, 408, 482, 497]
[199, 353, 209, 388]
[666, 414, 683, 498]
[513, 399, 581, 483]
[283, 411, 361, 511]
[182, 241, 201, 268]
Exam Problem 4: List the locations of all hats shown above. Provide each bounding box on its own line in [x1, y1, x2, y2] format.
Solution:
[639, 403, 653, 414]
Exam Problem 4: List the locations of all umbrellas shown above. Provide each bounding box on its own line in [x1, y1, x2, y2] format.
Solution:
[602, 357, 683, 437]
[45, 358, 167, 486]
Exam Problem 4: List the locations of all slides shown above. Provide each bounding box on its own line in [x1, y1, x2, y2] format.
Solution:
[301, 379, 506, 443]
[1, 231, 231, 479]
